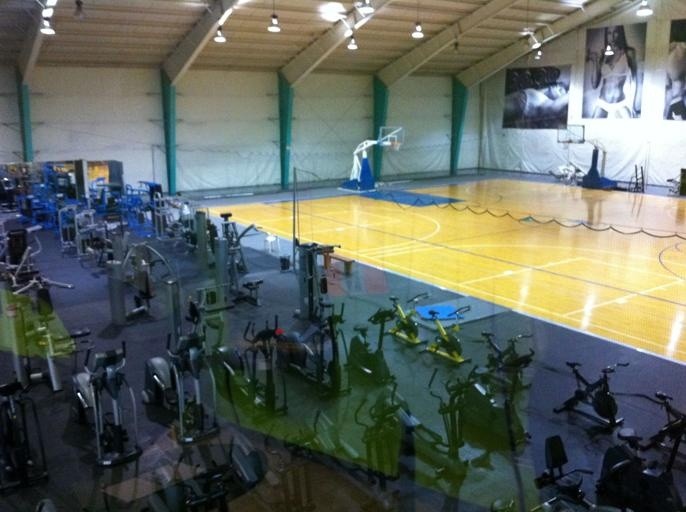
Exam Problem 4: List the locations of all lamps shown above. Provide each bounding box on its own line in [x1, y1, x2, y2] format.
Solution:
[525, 1, 653, 62]
[37, 1, 88, 36]
[214, 2, 463, 62]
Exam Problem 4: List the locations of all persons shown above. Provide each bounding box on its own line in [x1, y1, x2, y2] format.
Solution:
[504, 82, 569, 118]
[206, 220, 217, 254]
[169, 192, 184, 223]
[664, 41, 686, 121]
[589, 25, 643, 118]
